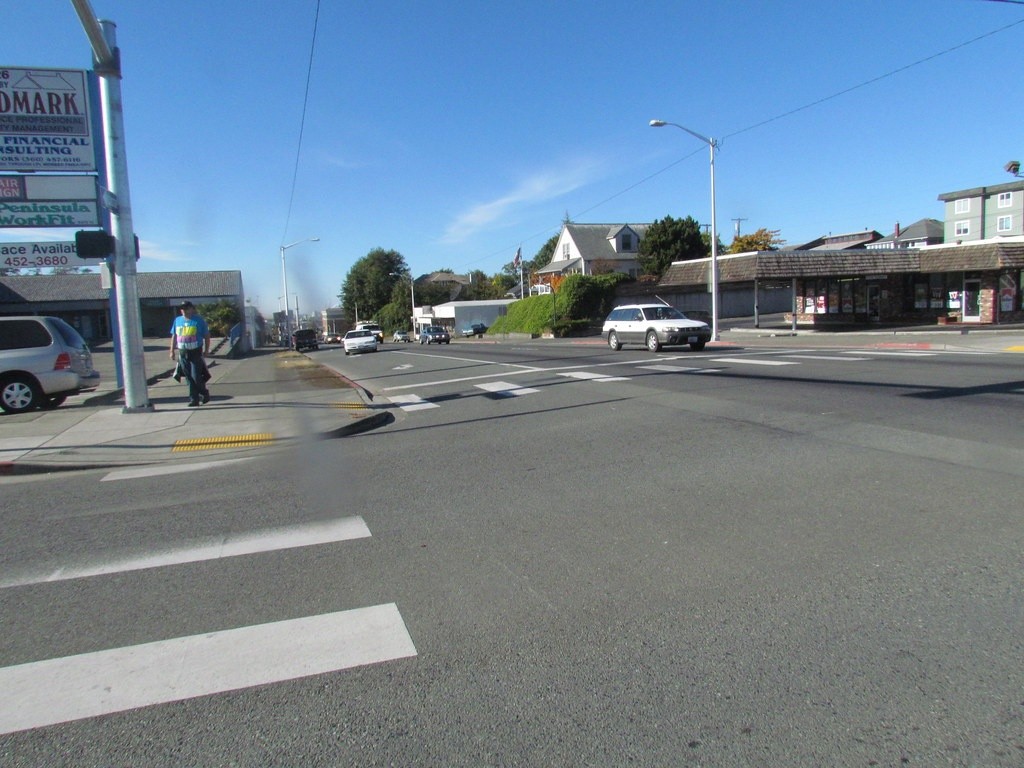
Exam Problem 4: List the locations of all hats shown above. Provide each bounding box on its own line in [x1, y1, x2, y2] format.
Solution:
[178, 301, 193, 308]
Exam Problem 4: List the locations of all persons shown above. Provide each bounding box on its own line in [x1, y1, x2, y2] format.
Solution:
[363, 331, 369, 336]
[637, 310, 642, 320]
[170, 301, 210, 407]
[436, 329, 439, 332]
[291, 334, 297, 350]
[662, 310, 670, 319]
[651, 309, 657, 319]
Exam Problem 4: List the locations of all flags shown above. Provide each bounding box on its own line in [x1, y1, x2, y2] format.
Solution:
[514, 247, 520, 268]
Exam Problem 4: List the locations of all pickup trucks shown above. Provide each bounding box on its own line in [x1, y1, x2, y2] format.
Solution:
[463, 323, 488, 338]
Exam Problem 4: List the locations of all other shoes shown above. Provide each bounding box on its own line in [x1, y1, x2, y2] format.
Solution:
[203, 392, 209, 404]
[188, 401, 199, 406]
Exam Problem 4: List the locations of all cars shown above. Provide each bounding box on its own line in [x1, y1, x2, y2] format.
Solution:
[393, 331, 410, 343]
[341, 329, 378, 355]
[323, 333, 342, 344]
[419, 326, 450, 345]
[601, 303, 712, 353]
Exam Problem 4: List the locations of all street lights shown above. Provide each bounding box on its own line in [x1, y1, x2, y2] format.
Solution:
[389, 272, 417, 341]
[539, 282, 556, 326]
[280, 236, 321, 350]
[649, 119, 720, 343]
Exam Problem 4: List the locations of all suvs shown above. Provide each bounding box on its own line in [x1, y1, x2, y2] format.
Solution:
[293, 330, 319, 351]
[356, 321, 383, 344]
[0, 315, 100, 413]
